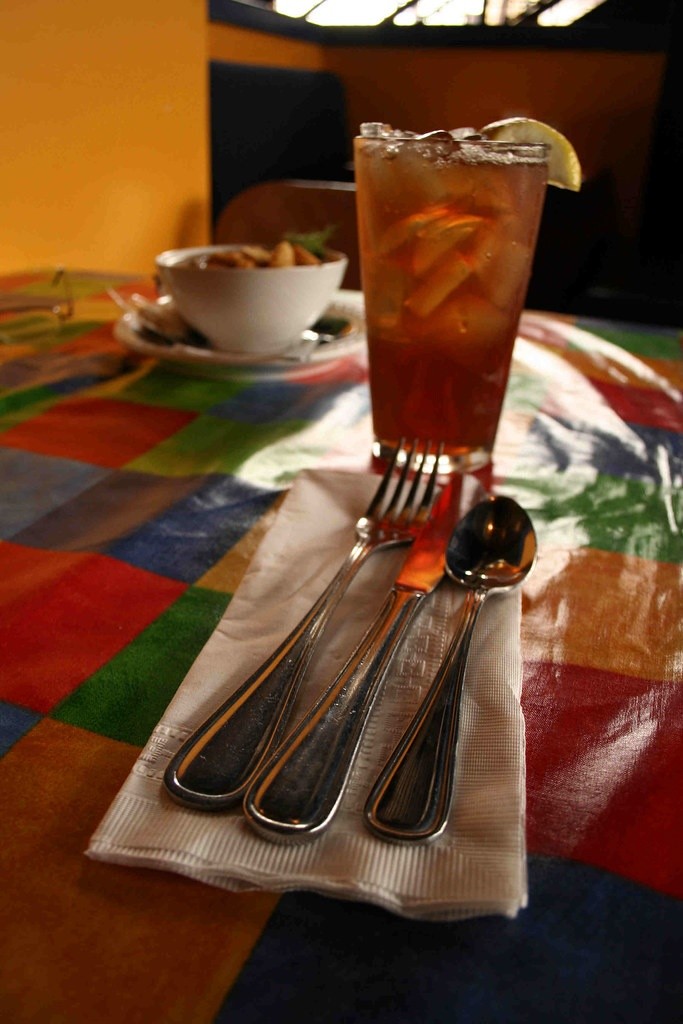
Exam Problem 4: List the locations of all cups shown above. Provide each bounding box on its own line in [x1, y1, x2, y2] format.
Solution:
[355, 134, 552, 474]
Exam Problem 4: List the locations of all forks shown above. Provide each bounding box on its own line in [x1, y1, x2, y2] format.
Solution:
[161, 436, 444, 810]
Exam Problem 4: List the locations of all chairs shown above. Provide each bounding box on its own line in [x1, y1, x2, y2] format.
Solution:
[215, 177, 361, 290]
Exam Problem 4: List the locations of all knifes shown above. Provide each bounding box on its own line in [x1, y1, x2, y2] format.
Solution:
[243, 473, 494, 845]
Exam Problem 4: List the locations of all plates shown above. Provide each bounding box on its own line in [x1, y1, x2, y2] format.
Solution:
[113, 294, 367, 382]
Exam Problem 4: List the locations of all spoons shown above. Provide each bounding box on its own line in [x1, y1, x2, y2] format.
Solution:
[363, 496, 537, 847]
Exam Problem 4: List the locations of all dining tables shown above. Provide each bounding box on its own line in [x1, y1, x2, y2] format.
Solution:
[0, 268, 682, 1024]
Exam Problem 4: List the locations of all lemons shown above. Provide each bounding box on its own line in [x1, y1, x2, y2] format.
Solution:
[477, 116, 580, 190]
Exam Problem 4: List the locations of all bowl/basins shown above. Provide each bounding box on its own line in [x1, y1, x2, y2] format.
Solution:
[155, 246, 348, 357]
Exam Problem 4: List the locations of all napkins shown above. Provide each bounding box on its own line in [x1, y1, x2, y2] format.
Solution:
[84, 465, 530, 921]
